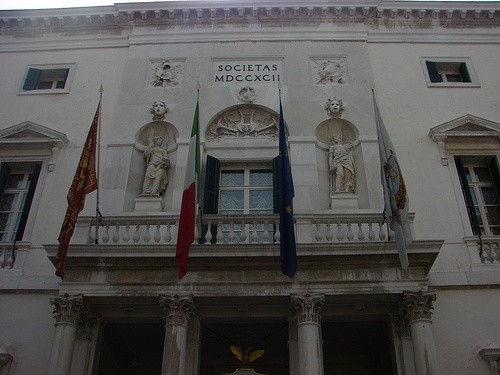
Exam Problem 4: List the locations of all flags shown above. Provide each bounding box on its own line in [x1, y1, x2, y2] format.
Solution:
[372, 88, 413, 272]
[175, 81, 202, 278]
[54, 92, 100, 280]
[278, 89, 298, 279]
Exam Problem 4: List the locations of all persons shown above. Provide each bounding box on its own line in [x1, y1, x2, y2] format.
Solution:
[329, 134, 356, 194]
[324, 96, 345, 119]
[150, 99, 169, 120]
[140, 135, 172, 197]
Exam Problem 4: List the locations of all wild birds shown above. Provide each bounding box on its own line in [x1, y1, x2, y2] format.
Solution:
[230, 344, 264, 366]
